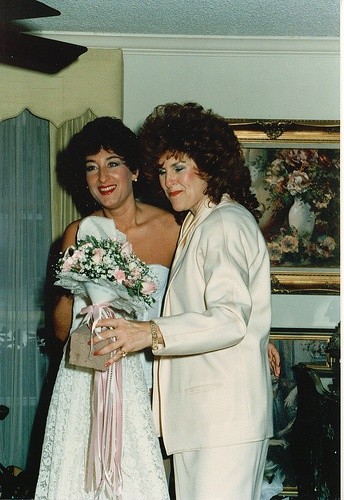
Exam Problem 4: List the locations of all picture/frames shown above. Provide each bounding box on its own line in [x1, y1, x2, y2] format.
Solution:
[260, 328, 341, 497]
[224, 118, 341, 296]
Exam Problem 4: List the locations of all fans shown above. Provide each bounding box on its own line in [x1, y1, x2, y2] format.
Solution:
[0, 1, 90, 79]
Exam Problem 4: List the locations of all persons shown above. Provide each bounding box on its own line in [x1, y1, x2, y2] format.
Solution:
[88, 103, 274, 500]
[35, 117, 281, 500]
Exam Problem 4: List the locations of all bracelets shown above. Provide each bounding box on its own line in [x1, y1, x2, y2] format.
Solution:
[149, 320, 159, 350]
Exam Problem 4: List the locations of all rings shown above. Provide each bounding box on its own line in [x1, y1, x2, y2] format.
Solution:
[120, 349, 127, 358]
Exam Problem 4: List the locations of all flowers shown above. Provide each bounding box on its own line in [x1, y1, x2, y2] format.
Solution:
[54, 233, 158, 296]
